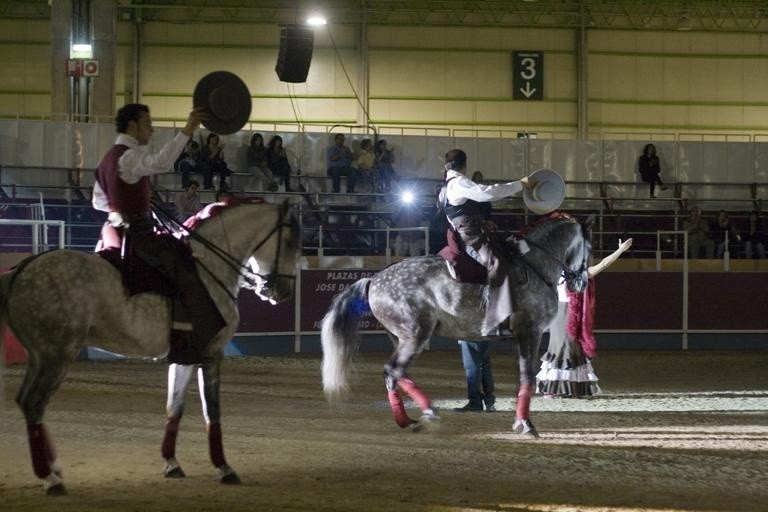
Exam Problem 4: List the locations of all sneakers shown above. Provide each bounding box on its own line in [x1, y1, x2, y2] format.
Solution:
[485, 404, 497, 413]
[660, 183, 668, 191]
[453, 399, 483, 412]
[649, 193, 657, 198]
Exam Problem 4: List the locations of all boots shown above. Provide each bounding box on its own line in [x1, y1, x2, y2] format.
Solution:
[495, 315, 515, 338]
[165, 327, 216, 367]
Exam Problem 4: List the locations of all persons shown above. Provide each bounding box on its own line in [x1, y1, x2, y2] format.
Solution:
[437, 149, 536, 333]
[454, 340, 496, 411]
[682, 206, 767, 258]
[535, 237, 633, 398]
[472, 171, 483, 183]
[639, 145, 668, 198]
[92, 104, 210, 364]
[176, 134, 424, 257]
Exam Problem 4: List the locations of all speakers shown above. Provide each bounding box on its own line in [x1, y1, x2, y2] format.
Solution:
[275, 29, 314, 83]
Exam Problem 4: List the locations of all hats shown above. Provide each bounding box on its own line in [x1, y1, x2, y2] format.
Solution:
[521, 166, 568, 218]
[190, 68, 253, 136]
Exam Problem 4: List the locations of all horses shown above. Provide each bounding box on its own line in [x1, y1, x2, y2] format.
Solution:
[317, 213, 598, 439]
[1, 198, 303, 497]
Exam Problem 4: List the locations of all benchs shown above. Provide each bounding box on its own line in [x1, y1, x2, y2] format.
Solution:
[1, 161, 451, 265]
[490, 161, 767, 264]
